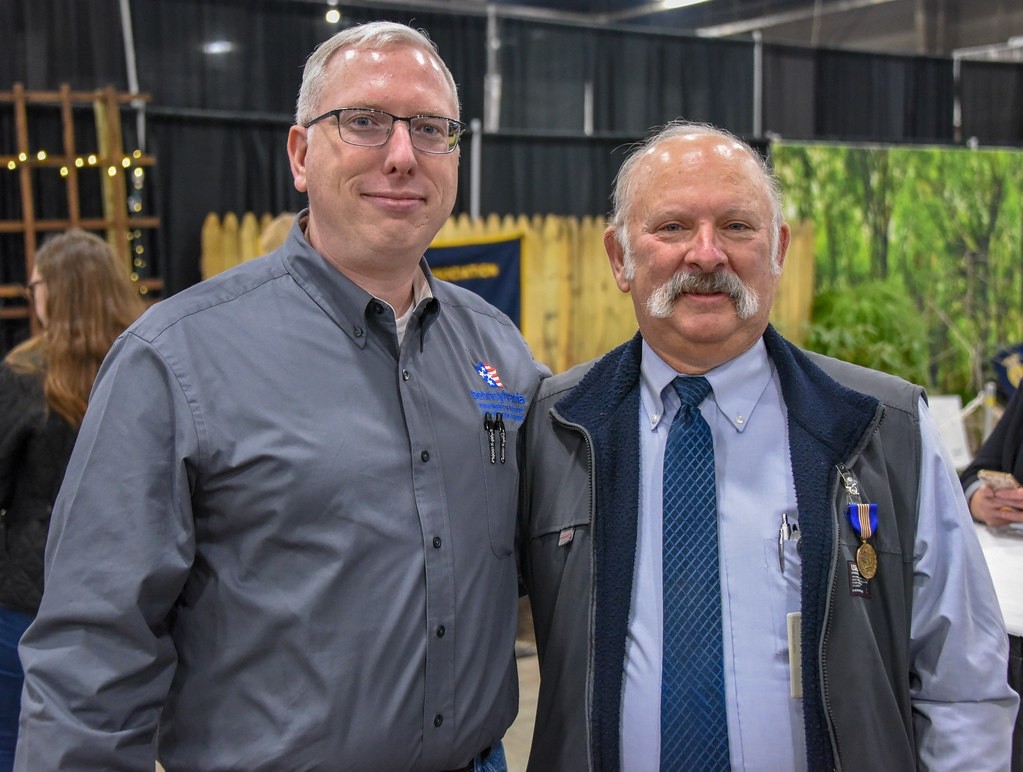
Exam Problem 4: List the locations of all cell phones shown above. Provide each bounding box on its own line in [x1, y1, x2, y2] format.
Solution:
[978, 470, 1023, 511]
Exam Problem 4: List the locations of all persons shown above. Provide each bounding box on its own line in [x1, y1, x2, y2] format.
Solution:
[958, 377, 1023, 528]
[516, 115, 1020, 772]
[12, 20, 551, 770]
[0, 233, 150, 771]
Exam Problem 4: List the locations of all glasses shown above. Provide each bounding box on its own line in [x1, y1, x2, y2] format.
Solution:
[25, 279, 48, 299]
[306, 108, 466, 154]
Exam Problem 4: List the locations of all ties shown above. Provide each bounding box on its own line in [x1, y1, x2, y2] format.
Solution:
[659, 377, 734, 771]
